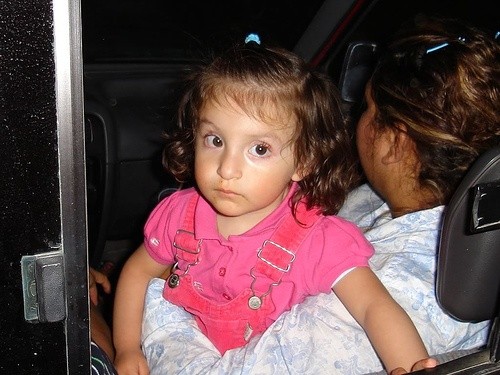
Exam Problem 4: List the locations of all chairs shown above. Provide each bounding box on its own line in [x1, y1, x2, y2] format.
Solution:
[396, 144, 500, 375]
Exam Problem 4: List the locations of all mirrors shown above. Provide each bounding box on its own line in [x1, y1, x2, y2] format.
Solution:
[341, 44, 377, 103]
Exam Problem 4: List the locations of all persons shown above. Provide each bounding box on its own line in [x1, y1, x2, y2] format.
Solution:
[111, 34, 437, 375]
[138, 23, 498, 375]
[87, 259, 117, 375]
